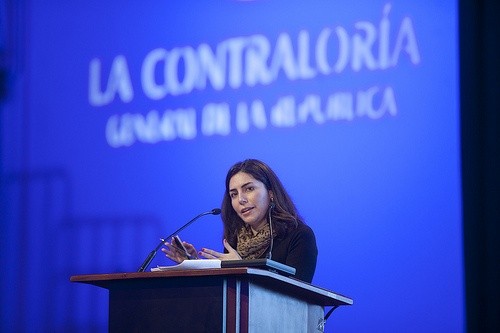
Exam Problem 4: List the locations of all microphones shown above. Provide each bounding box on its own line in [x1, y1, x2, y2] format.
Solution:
[138, 208, 221, 272]
[268, 202, 276, 259]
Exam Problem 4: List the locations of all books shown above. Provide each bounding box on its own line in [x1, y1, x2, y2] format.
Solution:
[151, 257, 222, 271]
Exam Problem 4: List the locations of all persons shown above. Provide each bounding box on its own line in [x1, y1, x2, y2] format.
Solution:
[161, 159, 318, 284]
